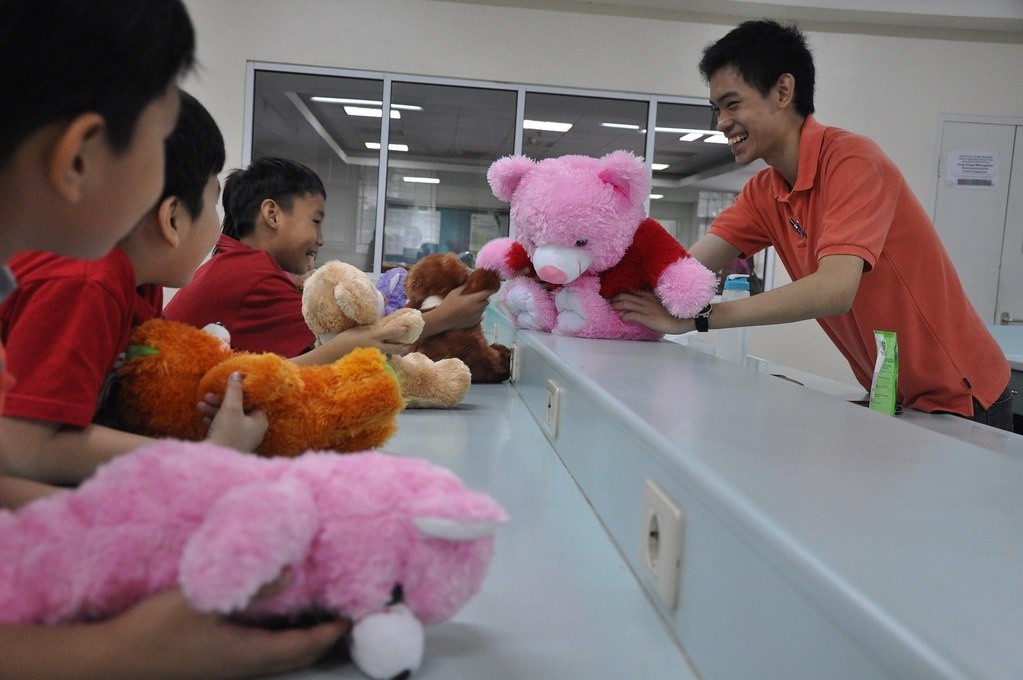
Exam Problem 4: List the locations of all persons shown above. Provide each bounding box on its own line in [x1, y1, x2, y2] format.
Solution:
[1, 0, 498, 680]
[515, 20, 1012, 433]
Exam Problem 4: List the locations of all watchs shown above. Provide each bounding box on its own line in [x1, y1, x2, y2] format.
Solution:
[693, 304, 712, 333]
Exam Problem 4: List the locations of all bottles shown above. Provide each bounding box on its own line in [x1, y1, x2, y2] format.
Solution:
[715, 275, 750, 366]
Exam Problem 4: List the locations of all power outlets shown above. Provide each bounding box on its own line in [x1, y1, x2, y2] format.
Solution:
[635, 479, 686, 612]
[509, 342, 517, 382]
[481, 311, 498, 344]
[544, 379, 560, 438]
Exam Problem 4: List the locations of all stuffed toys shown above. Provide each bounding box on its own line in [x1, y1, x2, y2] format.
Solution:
[2, 149, 716, 679]
